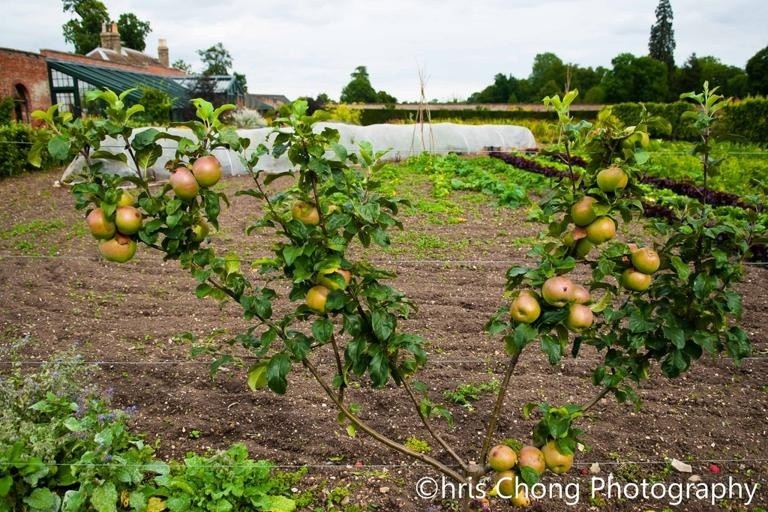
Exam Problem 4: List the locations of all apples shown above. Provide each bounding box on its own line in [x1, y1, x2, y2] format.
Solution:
[86, 188, 142, 262]
[489, 440, 577, 506]
[168, 167, 199, 202]
[191, 155, 223, 188]
[306, 285, 331, 317]
[293, 202, 320, 225]
[565, 166, 629, 257]
[509, 276, 593, 335]
[623, 126, 649, 150]
[192, 221, 208, 241]
[316, 269, 351, 293]
[621, 244, 660, 291]
